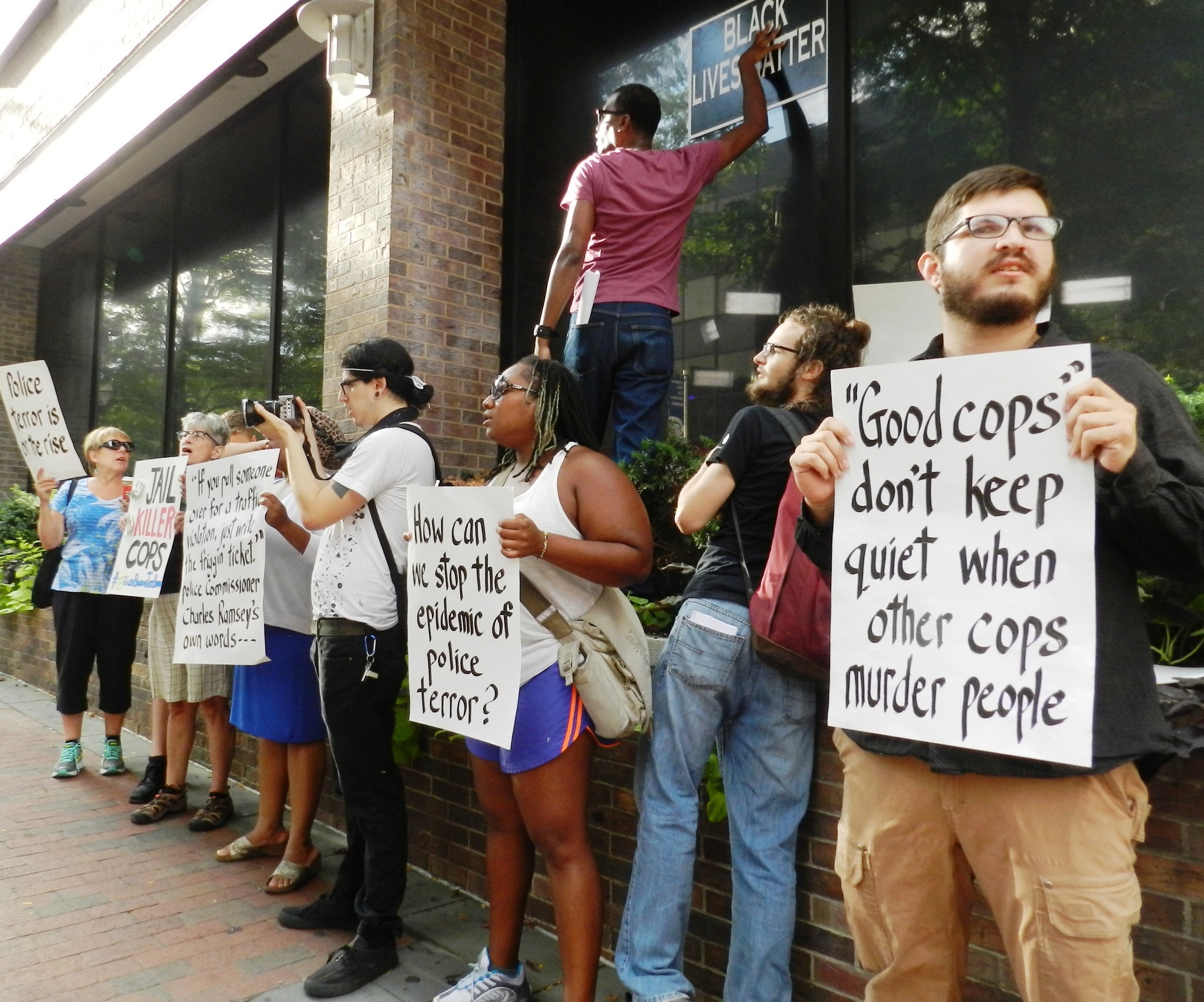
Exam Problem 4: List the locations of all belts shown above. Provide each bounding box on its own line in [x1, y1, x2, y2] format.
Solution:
[309, 617, 400, 637]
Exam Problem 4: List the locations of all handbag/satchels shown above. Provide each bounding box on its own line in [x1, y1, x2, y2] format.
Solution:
[30, 479, 78, 609]
[558, 582, 655, 754]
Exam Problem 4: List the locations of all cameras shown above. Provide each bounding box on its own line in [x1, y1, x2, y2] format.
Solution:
[242, 395, 300, 429]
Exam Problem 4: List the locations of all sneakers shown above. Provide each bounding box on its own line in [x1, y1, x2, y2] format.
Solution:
[186, 794, 234, 831]
[277, 891, 360, 928]
[303, 935, 400, 997]
[432, 946, 532, 1002]
[131, 786, 189, 824]
[52, 742, 84, 777]
[100, 738, 125, 775]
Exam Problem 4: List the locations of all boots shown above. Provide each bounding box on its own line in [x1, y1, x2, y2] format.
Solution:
[129, 755, 167, 804]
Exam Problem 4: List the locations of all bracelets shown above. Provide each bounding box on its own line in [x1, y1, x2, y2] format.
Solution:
[533, 530, 548, 560]
[532, 323, 556, 341]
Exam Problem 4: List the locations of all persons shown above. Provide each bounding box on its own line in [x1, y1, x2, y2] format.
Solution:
[403, 353, 659, 1002]
[530, 19, 786, 485]
[612, 299, 873, 1002]
[785, 142, 1203, 1002]
[127, 408, 272, 814]
[255, 336, 446, 1000]
[30, 424, 146, 779]
[129, 411, 237, 836]
[211, 405, 353, 896]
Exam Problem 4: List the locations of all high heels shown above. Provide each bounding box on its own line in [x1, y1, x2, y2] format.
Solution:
[214, 835, 289, 862]
[262, 852, 322, 893]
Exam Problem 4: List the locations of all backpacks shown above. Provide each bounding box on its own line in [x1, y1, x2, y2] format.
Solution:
[725, 405, 832, 682]
[365, 421, 455, 643]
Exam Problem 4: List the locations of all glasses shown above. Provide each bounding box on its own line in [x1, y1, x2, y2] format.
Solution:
[932, 213, 1063, 255]
[93, 439, 135, 452]
[176, 430, 220, 446]
[763, 343, 801, 360]
[337, 377, 363, 395]
[489, 374, 544, 401]
[595, 108, 629, 124]
